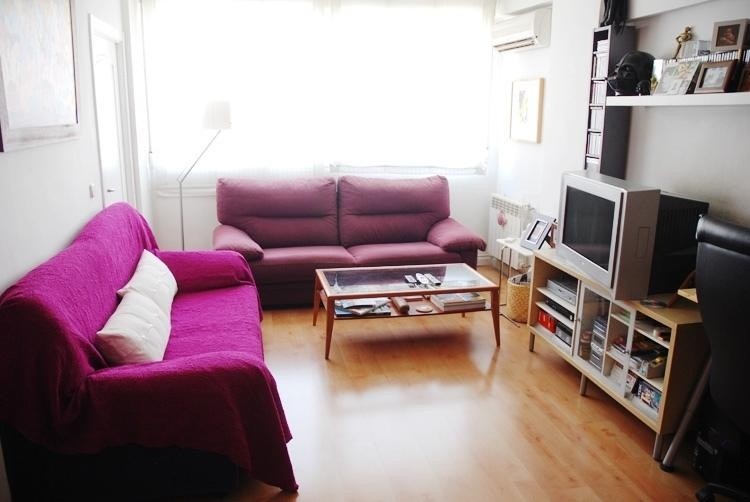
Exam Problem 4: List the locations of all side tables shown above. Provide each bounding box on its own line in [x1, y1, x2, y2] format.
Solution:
[496, 239, 550, 328]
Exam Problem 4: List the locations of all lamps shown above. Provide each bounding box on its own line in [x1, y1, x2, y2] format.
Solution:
[176, 100, 232, 251]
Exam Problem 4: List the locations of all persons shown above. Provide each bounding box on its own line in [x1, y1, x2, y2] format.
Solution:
[717, 27, 736, 45]
[672, 27, 692, 58]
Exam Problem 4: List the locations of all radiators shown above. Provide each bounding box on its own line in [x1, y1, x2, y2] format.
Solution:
[487, 193, 531, 271]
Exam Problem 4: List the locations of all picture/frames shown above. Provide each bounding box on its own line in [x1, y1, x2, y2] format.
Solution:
[508, 78, 545, 143]
[0, 1, 84, 155]
[693, 59, 738, 92]
[711, 18, 747, 52]
[520, 213, 556, 250]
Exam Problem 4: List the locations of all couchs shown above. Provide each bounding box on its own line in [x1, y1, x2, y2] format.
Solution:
[213, 176, 486, 311]
[3, 202, 299, 495]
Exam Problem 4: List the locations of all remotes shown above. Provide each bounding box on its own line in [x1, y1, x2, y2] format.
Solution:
[416, 273, 429, 284]
[405, 275, 417, 283]
[424, 273, 441, 285]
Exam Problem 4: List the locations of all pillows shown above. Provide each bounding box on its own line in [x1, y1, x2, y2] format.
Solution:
[95, 293, 172, 364]
[116, 249, 179, 321]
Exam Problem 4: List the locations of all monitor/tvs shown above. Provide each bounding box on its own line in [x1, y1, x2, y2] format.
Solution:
[556, 170, 710, 299]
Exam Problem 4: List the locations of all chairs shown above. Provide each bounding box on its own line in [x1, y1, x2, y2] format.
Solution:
[659, 214, 749, 496]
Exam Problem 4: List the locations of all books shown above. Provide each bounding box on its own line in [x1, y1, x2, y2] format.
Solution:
[608, 336, 664, 372]
[334, 292, 486, 318]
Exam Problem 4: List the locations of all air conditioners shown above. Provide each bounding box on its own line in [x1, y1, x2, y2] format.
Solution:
[491, 8, 551, 54]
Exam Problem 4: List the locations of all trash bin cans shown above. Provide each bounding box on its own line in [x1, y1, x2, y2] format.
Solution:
[506, 274, 530, 323]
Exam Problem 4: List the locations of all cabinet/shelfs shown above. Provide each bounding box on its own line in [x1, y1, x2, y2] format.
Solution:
[525, 249, 709, 463]
[584, 21, 637, 179]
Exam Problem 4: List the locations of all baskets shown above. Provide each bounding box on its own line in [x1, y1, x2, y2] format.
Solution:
[505, 271, 532, 325]
